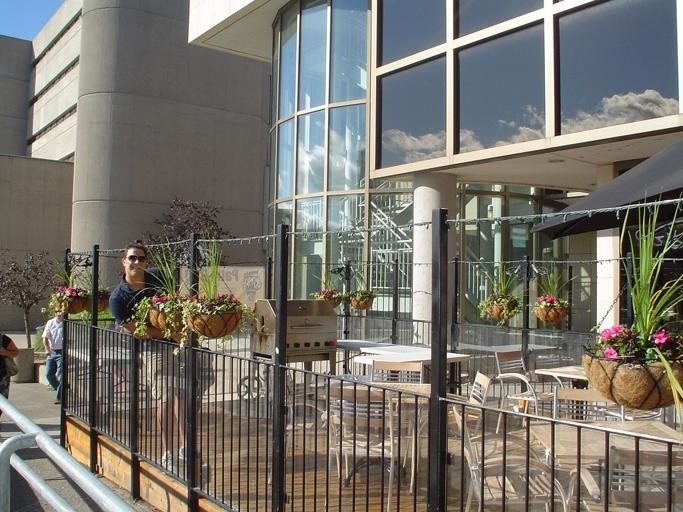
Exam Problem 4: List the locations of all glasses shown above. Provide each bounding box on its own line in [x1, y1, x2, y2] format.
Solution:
[125, 255, 147, 262]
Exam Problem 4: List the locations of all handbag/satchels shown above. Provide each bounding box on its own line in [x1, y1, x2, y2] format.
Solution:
[1, 347, 18, 377]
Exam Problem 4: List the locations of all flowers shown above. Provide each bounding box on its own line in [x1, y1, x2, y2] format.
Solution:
[308, 264, 380, 305]
[41, 235, 265, 357]
[476, 258, 580, 329]
[583, 184, 682, 449]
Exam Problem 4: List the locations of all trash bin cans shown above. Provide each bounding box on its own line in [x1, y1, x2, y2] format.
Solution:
[9, 347, 36, 383]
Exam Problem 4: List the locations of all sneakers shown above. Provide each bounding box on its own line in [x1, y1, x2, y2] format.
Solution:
[161, 450, 173, 471]
[55, 399, 62, 403]
[179, 447, 188, 460]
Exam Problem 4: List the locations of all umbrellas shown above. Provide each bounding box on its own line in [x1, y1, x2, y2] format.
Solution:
[530, 137, 682, 242]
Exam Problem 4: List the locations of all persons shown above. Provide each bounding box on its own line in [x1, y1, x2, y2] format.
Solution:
[0, 333, 19, 418]
[107, 241, 215, 476]
[40, 302, 63, 404]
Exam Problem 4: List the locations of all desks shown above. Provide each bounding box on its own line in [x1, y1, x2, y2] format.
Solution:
[359, 345, 470, 398]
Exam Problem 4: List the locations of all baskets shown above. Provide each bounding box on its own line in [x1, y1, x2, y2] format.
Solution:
[582, 350, 683, 409]
[187, 312, 241, 339]
[149, 309, 182, 330]
[350, 297, 372, 310]
[533, 307, 567, 321]
[89, 293, 109, 312]
[56, 295, 88, 314]
[321, 296, 342, 307]
[487, 303, 515, 319]
